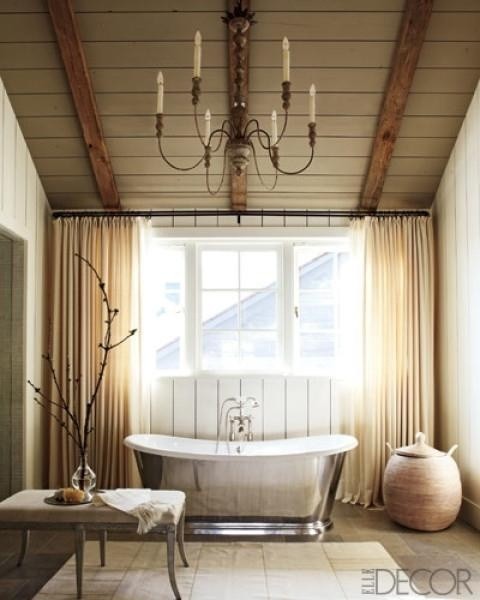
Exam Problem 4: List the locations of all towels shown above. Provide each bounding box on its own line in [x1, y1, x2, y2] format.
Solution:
[91, 485, 175, 536]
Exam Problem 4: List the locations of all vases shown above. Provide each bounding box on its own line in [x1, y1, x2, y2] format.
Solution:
[71, 455, 97, 492]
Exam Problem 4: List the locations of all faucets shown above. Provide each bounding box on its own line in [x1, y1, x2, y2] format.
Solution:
[236, 422, 246, 436]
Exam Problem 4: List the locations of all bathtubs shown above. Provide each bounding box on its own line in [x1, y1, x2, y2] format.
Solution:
[122, 434, 359, 522]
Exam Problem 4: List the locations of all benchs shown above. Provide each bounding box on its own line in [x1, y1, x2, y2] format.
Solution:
[0, 488, 190, 600]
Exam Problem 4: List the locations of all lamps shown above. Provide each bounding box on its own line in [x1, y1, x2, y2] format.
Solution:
[155, 4, 318, 197]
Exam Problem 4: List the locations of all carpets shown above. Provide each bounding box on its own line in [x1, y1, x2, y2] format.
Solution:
[33, 539, 426, 600]
